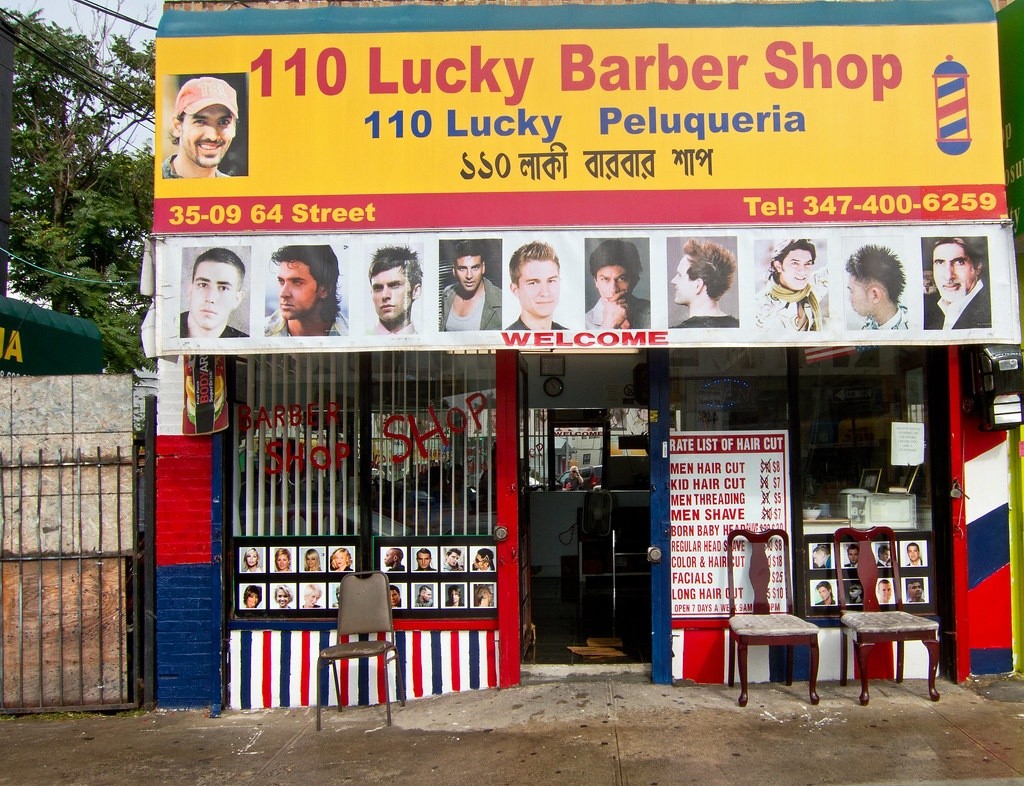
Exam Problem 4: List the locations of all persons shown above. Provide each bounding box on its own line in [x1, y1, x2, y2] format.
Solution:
[564, 465, 584, 491]
[265, 244, 350, 337]
[438, 239, 502, 331]
[667, 237, 740, 328]
[504, 236, 572, 331]
[922, 235, 993, 331]
[162, 70, 248, 179]
[238, 541, 499, 610]
[803, 535, 932, 608]
[841, 236, 917, 331]
[754, 239, 832, 331]
[584, 237, 651, 330]
[364, 242, 427, 334]
[180, 246, 251, 338]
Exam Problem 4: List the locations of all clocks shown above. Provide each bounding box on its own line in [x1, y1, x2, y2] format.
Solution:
[543, 377, 564, 397]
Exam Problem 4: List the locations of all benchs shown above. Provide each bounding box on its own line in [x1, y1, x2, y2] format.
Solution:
[575, 507, 651, 615]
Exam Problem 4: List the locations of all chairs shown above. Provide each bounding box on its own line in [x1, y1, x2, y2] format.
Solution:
[727, 527, 820, 706]
[834, 526, 941, 706]
[316, 571, 406, 731]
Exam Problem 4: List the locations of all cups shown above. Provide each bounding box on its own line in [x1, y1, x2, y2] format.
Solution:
[813, 504, 831, 518]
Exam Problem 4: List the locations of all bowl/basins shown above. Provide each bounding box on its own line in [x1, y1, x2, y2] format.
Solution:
[803, 510, 822, 520]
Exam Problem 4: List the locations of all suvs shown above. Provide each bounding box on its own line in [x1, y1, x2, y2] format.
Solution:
[557, 465, 602, 492]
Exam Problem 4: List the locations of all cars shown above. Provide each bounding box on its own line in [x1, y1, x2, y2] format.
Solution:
[529, 477, 549, 493]
[240, 503, 421, 537]
[411, 491, 434, 505]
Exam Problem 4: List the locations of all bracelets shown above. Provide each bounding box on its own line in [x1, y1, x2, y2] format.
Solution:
[577, 474, 580, 476]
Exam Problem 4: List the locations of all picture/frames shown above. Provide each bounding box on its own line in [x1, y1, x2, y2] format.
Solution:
[858, 468, 883, 493]
